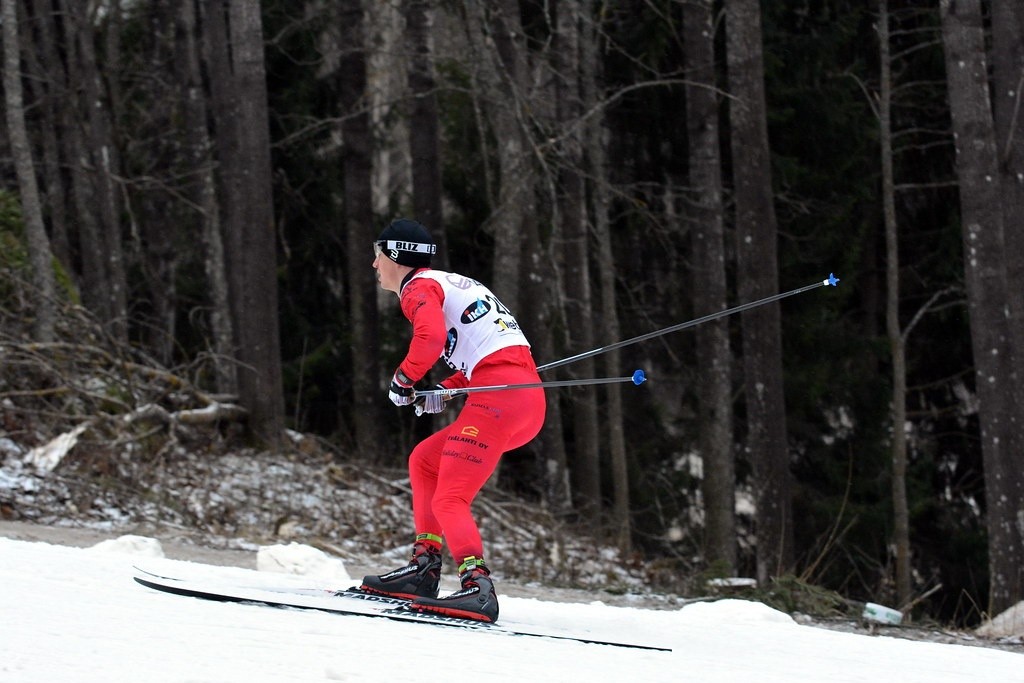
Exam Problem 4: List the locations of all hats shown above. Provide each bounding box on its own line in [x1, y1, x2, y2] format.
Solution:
[376, 218, 436, 268]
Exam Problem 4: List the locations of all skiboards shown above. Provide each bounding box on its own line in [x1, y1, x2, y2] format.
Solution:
[132, 562, 676, 653]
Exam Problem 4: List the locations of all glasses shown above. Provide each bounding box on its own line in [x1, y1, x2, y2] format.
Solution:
[372, 239, 385, 258]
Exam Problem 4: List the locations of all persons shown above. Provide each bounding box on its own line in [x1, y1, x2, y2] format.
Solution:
[359, 221, 546, 624]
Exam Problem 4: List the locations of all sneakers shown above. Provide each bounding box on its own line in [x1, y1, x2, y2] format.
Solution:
[408, 560, 500, 622]
[361, 537, 443, 600]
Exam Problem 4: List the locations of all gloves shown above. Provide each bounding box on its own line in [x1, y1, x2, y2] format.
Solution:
[414, 383, 447, 416]
[388, 367, 416, 407]
